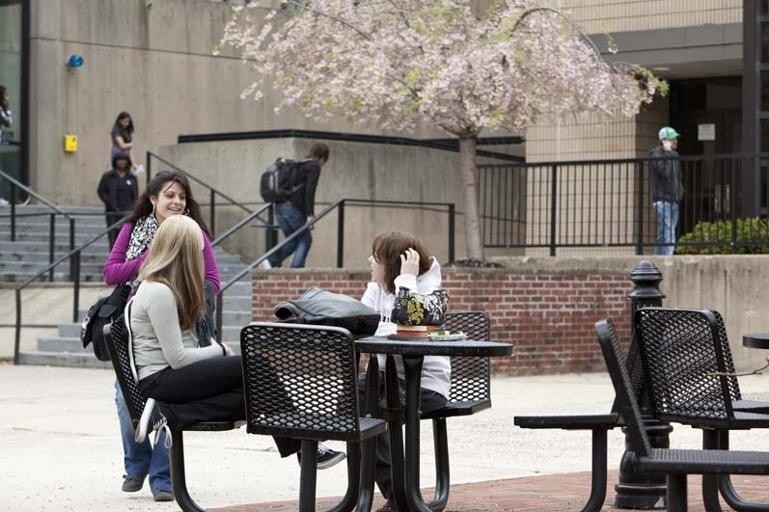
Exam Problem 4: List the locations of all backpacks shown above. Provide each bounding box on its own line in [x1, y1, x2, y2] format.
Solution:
[258, 156, 315, 204]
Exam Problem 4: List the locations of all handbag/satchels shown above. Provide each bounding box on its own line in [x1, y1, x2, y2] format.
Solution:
[276, 289, 381, 339]
[80, 284, 129, 363]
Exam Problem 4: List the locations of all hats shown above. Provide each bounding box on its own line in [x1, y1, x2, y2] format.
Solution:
[658, 127, 682, 140]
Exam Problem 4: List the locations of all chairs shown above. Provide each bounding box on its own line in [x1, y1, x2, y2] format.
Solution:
[630, 306, 769, 512]
[105, 316, 256, 512]
[364, 312, 493, 511]
[237, 322, 388, 512]
[592, 319, 769, 512]
[515, 311, 665, 512]
[651, 308, 769, 512]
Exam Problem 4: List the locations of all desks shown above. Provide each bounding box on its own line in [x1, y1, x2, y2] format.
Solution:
[742, 335, 769, 350]
[241, 328, 513, 512]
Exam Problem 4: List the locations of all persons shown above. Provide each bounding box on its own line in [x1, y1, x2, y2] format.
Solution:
[97, 151, 139, 252]
[109, 110, 140, 171]
[647, 125, 686, 255]
[124, 213, 346, 471]
[105, 169, 222, 501]
[0, 84, 14, 206]
[343, 230, 454, 510]
[257, 141, 331, 268]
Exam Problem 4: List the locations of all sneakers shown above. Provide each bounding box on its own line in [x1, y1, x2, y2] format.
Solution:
[121, 468, 150, 491]
[298, 444, 345, 470]
[152, 481, 174, 501]
